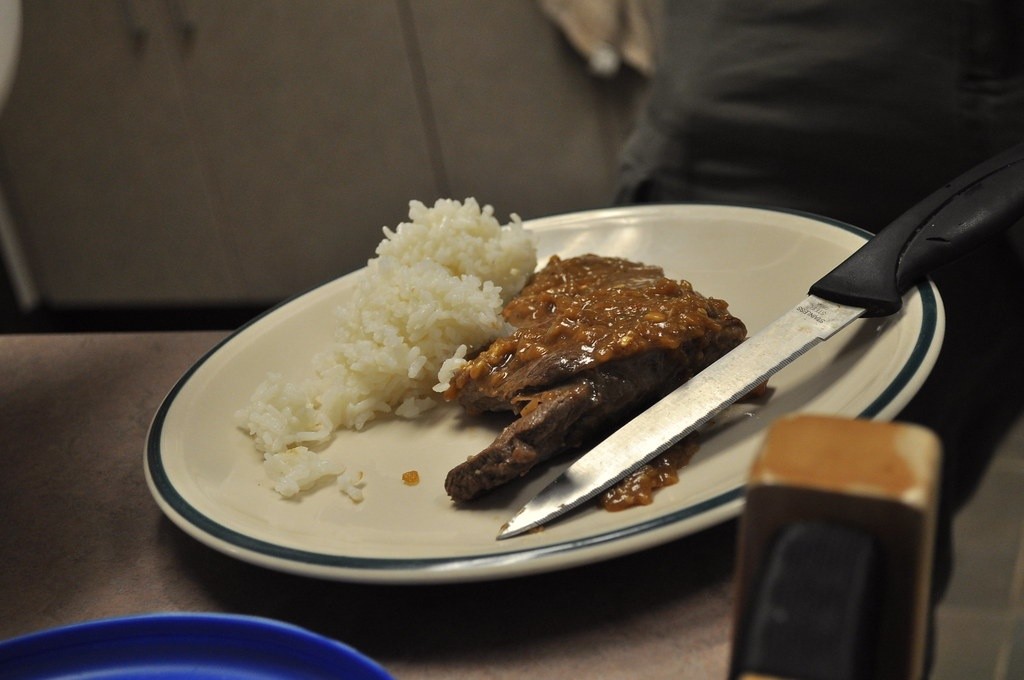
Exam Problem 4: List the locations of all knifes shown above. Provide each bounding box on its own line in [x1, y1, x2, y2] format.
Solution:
[494, 137, 1024, 542]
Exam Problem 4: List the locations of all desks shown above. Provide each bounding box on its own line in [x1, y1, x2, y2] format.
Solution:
[0, 334, 1022, 679]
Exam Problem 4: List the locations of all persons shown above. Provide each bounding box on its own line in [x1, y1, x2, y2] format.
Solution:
[614, 0, 1024, 680]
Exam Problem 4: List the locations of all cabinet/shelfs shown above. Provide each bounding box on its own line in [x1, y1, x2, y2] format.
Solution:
[1, 0, 666, 316]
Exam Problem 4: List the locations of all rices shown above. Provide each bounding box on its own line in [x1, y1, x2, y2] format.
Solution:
[231, 195, 540, 501]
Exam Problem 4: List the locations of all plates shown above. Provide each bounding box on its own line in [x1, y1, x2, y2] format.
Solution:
[141, 200, 947, 583]
